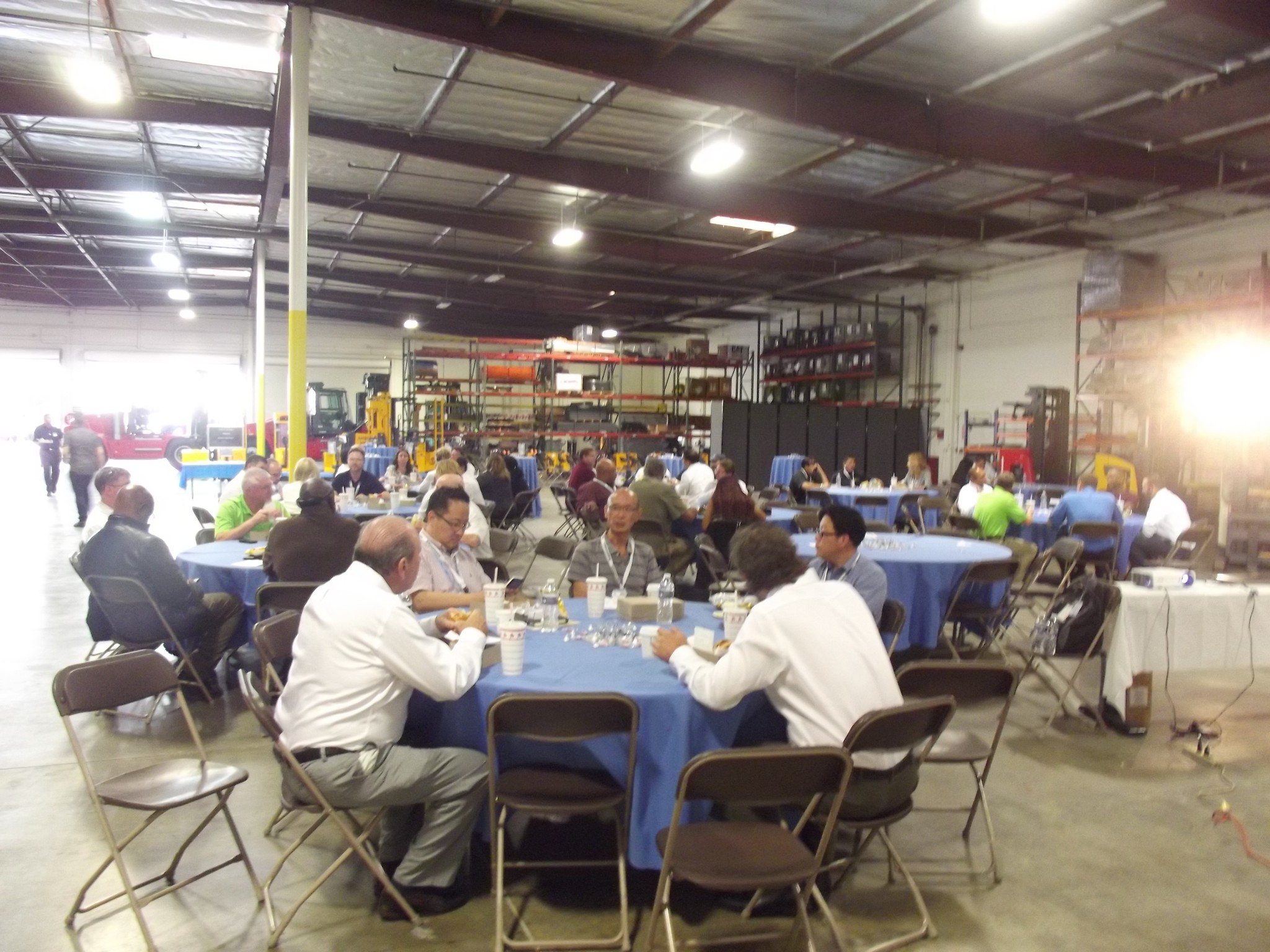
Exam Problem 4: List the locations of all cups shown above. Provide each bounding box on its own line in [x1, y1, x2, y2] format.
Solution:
[399, 488, 408, 498]
[891, 476, 898, 486]
[1026, 499, 1035, 513]
[723, 607, 747, 641]
[499, 620, 526, 674]
[340, 492, 348, 512]
[388, 476, 396, 484]
[646, 584, 660, 598]
[391, 492, 400, 509]
[275, 517, 288, 524]
[1014, 493, 1024, 508]
[586, 576, 607, 619]
[483, 583, 505, 626]
[1116, 499, 1124, 512]
[346, 487, 354, 500]
[410, 472, 417, 481]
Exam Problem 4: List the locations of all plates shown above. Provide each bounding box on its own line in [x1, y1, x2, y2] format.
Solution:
[713, 610, 724, 619]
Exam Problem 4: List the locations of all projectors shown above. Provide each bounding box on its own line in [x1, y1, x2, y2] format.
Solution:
[1131, 565, 1186, 588]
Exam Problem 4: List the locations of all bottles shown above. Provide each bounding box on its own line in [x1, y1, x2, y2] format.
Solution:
[541, 578, 558, 632]
[657, 573, 675, 624]
[836, 472, 841, 487]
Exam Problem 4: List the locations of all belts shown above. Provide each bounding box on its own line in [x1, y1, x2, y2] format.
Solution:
[286, 747, 357, 768]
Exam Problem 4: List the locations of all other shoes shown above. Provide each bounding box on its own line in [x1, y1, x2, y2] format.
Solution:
[378, 878, 472, 922]
[373, 858, 407, 898]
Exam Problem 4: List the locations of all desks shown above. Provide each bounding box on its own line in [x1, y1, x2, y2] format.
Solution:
[280, 500, 427, 529]
[403, 592, 785, 876]
[646, 452, 696, 478]
[176, 539, 268, 625]
[1012, 483, 1072, 501]
[670, 498, 803, 546]
[784, 531, 1012, 668]
[366, 442, 542, 517]
[1102, 575, 1270, 724]
[808, 482, 945, 538]
[1013, 507, 1150, 577]
[770, 451, 808, 496]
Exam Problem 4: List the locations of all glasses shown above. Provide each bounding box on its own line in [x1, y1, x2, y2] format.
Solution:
[296, 491, 333, 508]
[607, 504, 638, 514]
[435, 511, 471, 530]
[815, 527, 836, 538]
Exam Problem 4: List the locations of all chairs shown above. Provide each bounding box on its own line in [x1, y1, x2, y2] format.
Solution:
[237, 663, 431, 948]
[252, 578, 328, 725]
[645, 745, 856, 952]
[52, 654, 263, 947]
[874, 659, 1023, 884]
[488, 691, 637, 951]
[253, 608, 391, 853]
[1064, 519, 1121, 585]
[1146, 521, 1217, 571]
[925, 561, 1019, 666]
[991, 534, 1083, 667]
[879, 599, 908, 660]
[1009, 581, 1122, 732]
[750, 476, 838, 534]
[69, 504, 223, 663]
[852, 492, 990, 540]
[476, 465, 750, 611]
[741, 698, 956, 952]
[88, 572, 215, 718]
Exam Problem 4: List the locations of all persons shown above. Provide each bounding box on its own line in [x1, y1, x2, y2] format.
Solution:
[32, 414, 63, 498]
[647, 521, 919, 922]
[1102, 465, 1139, 514]
[1126, 472, 1196, 576]
[255, 476, 363, 651]
[1045, 472, 1125, 588]
[77, 484, 241, 700]
[81, 463, 137, 550]
[788, 455, 830, 508]
[972, 471, 1038, 595]
[803, 505, 888, 628]
[830, 456, 862, 486]
[62, 410, 106, 528]
[210, 439, 776, 600]
[955, 468, 993, 523]
[951, 455, 995, 492]
[896, 451, 933, 527]
[272, 514, 496, 921]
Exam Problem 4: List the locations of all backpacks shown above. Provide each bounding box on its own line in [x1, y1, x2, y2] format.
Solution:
[1046, 575, 1106, 654]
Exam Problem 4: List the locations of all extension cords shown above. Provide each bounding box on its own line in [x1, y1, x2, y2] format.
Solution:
[1185, 746, 1220, 768]
